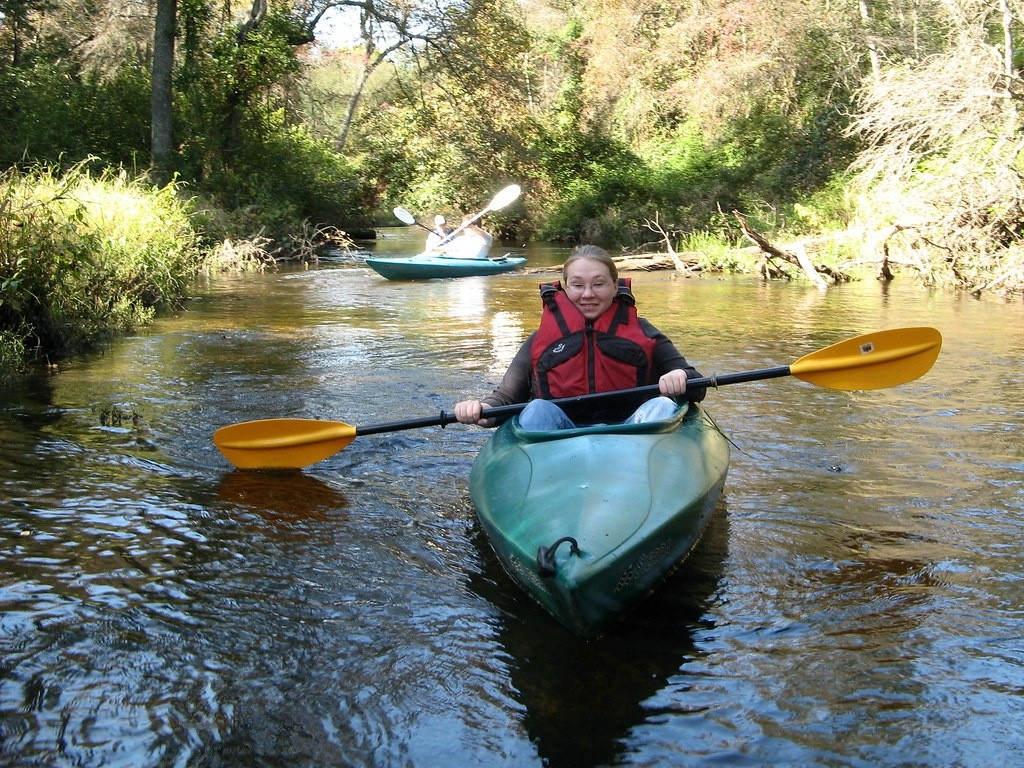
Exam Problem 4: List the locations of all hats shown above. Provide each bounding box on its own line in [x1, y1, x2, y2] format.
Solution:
[434, 215, 446, 226]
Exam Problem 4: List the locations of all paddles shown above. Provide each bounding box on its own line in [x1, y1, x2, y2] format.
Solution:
[430, 184, 521, 248]
[214, 326, 943, 468]
[393, 207, 445, 239]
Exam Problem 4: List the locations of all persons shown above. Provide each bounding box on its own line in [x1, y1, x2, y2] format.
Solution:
[454, 244, 707, 432]
[426, 214, 455, 250]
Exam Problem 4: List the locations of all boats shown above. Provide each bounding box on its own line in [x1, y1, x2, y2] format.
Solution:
[468, 402, 730, 641]
[364, 253, 529, 281]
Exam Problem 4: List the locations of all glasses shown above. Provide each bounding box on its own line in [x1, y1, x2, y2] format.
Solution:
[565, 278, 616, 292]
